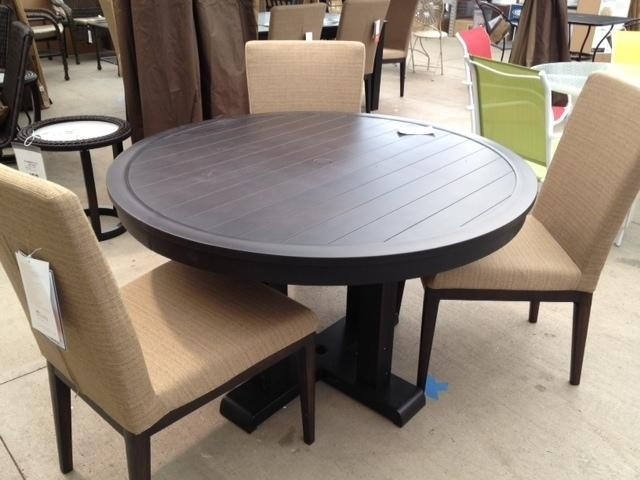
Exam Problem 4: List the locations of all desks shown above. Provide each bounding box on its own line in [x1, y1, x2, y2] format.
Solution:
[105, 109, 539, 436]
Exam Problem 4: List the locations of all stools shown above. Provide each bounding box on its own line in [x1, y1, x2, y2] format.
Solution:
[22, 71, 42, 125]
[16, 115, 134, 243]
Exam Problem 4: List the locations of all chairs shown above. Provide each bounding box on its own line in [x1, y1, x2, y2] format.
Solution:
[24, 2, 121, 80]
[243, 0, 415, 114]
[0, 163, 322, 480]
[393, 72, 640, 393]
[408, 1, 639, 246]
[0, 4, 35, 161]
